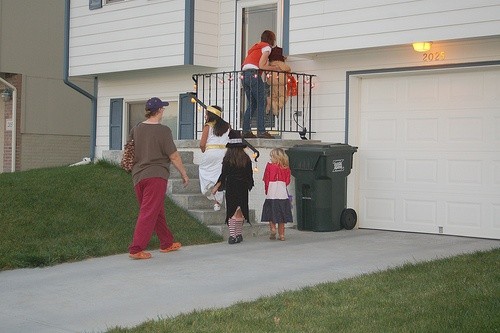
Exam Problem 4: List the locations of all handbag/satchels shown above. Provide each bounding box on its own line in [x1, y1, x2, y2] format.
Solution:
[120, 140, 135, 168]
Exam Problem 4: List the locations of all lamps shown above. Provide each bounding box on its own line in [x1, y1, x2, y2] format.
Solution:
[0, 87, 12, 103]
[412, 41, 433, 52]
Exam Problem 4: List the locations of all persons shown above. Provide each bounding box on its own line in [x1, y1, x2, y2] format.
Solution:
[198, 105, 229, 210]
[240, 30, 281, 139]
[211, 131, 254, 245]
[261, 149, 293, 241]
[121, 98, 189, 259]
[265, 46, 291, 116]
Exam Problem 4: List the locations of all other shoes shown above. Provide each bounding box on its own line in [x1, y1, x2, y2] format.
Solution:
[214, 204, 221, 211]
[128, 251, 151, 259]
[160, 243, 181, 252]
[278, 234, 284, 241]
[229, 235, 242, 244]
[269, 229, 276, 240]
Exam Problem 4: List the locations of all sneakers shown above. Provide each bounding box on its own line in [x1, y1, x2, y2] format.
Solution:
[242, 131, 256, 138]
[257, 131, 275, 139]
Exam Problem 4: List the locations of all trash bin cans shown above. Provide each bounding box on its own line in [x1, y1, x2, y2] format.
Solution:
[285, 143, 357, 231]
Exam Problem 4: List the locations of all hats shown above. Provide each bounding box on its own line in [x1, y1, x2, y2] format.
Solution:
[146, 97, 169, 109]
[225, 130, 248, 148]
